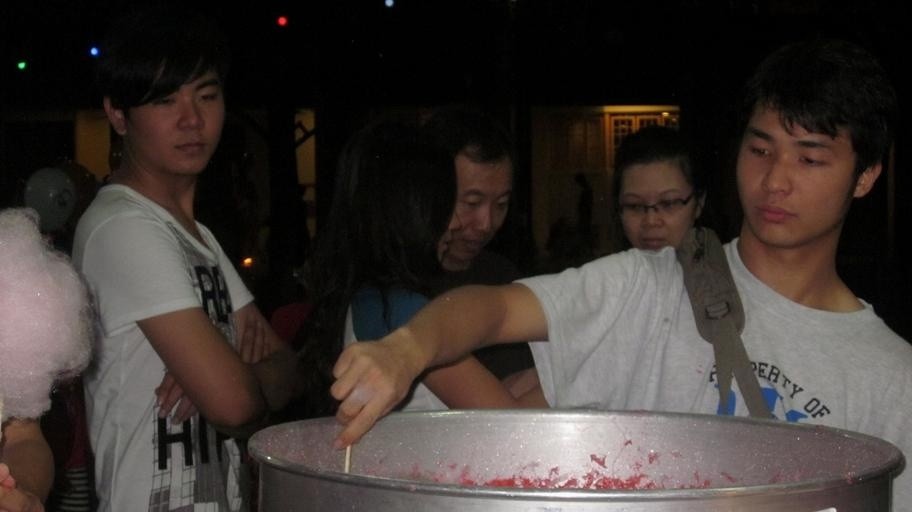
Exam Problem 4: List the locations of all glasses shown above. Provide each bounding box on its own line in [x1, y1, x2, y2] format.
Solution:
[615, 194, 693, 218]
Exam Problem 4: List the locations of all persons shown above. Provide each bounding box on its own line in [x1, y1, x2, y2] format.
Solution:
[418, 111, 544, 399]
[607, 125, 712, 253]
[325, 36, 912, 512]
[69, 22, 307, 510]
[0, 123, 313, 512]
[314, 108, 549, 415]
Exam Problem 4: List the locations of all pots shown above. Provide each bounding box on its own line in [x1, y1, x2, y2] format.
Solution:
[246, 401, 907, 512]
